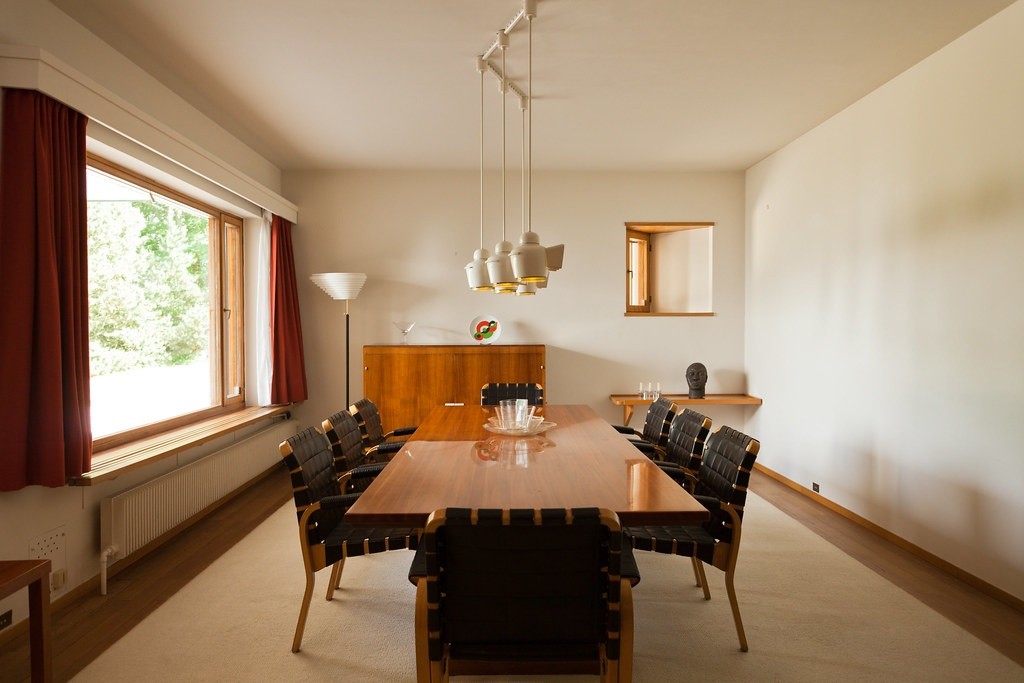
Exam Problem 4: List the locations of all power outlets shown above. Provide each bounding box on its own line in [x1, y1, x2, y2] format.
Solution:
[0, 609, 12, 630]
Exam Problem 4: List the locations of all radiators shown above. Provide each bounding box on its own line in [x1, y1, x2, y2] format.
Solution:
[101, 419, 300, 560]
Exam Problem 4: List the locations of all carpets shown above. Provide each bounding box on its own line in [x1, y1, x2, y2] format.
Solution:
[69, 498, 1023, 683]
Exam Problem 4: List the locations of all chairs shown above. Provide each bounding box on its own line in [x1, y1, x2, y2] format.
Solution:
[349, 398, 420, 461]
[613, 397, 679, 461]
[632, 408, 713, 491]
[321, 409, 405, 493]
[481, 383, 544, 407]
[625, 425, 761, 653]
[280, 426, 424, 653]
[408, 506, 641, 683]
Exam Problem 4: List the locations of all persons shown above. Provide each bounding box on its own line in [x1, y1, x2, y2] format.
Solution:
[686, 363, 708, 399]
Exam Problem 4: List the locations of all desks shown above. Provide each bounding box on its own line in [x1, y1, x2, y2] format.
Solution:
[344, 405, 710, 543]
[609, 394, 762, 427]
[0, 560, 54, 683]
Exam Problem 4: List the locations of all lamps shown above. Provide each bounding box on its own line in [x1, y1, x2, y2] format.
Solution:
[485, 29, 520, 294]
[508, 0, 565, 297]
[310, 272, 368, 410]
[464, 54, 495, 291]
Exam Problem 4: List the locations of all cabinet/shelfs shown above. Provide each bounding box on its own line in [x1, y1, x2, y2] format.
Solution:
[362, 344, 546, 457]
